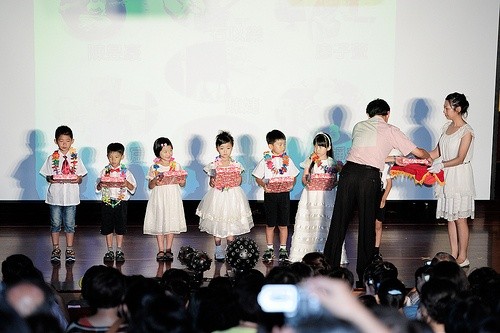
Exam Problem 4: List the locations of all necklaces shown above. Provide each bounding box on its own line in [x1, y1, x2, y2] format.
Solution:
[152, 156, 175, 179]
[263, 149, 290, 175]
[309, 152, 343, 175]
[52, 147, 78, 175]
[214, 155, 236, 192]
[100, 164, 128, 208]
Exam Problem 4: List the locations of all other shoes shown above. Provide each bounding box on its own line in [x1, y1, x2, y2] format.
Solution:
[372, 253, 382, 264]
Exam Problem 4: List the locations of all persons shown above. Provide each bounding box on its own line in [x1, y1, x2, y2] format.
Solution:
[418, 91, 477, 269]
[322, 98, 436, 289]
[285, 130, 349, 266]
[250, 130, 301, 264]
[38, 126, 88, 263]
[194, 130, 256, 262]
[0, 252, 500, 333]
[94, 143, 136, 262]
[143, 137, 188, 261]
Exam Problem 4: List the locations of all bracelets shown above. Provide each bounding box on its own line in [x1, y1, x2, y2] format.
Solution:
[393, 156, 397, 164]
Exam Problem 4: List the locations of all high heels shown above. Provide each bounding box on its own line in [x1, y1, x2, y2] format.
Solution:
[166, 252, 173, 261]
[457, 258, 471, 269]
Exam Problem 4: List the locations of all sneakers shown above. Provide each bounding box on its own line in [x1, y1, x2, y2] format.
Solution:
[115, 250, 125, 262]
[278, 248, 288, 259]
[103, 250, 114, 262]
[214, 245, 225, 260]
[260, 248, 276, 262]
[50, 248, 61, 262]
[65, 248, 76, 262]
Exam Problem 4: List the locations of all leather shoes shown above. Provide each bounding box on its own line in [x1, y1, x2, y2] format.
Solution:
[156, 251, 164, 261]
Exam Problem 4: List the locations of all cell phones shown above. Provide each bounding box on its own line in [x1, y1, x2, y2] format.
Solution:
[257, 283, 325, 315]
